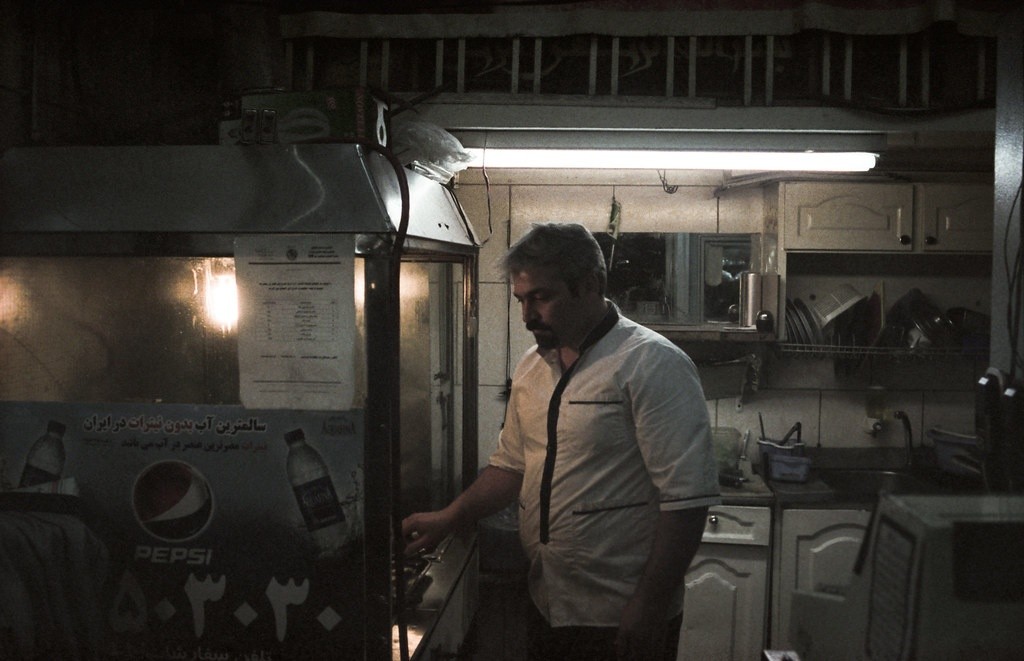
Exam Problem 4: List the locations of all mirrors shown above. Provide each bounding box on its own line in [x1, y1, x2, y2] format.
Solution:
[593, 230, 762, 326]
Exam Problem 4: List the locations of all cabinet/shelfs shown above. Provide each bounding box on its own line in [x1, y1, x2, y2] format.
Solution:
[763, 177, 993, 256]
[677, 505, 770, 661]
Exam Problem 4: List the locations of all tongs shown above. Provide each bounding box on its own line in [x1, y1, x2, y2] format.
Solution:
[778, 421, 801, 446]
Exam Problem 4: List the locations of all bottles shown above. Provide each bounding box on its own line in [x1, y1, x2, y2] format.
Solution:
[284, 428, 352, 554]
[18, 420, 66, 492]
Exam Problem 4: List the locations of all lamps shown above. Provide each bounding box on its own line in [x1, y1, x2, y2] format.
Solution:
[454, 130, 890, 172]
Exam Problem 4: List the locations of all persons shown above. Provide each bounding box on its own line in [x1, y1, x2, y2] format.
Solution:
[403, 227, 721, 661]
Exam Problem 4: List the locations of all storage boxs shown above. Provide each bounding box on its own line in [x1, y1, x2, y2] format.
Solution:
[240, 86, 392, 151]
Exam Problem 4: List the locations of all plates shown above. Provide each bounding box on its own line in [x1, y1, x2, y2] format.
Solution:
[786, 297, 825, 359]
